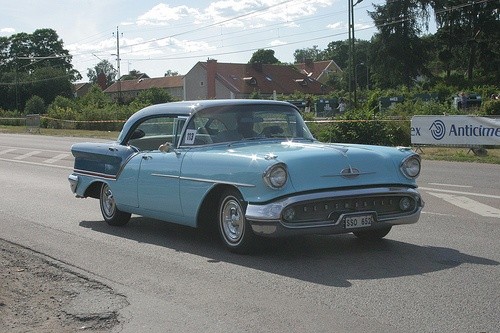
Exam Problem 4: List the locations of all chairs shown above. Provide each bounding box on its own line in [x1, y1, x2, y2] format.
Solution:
[130, 128, 241, 149]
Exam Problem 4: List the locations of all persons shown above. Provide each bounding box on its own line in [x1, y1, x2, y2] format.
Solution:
[460, 92, 469, 110]
[304, 94, 312, 113]
[159, 119, 204, 152]
[490, 94, 500, 100]
[336, 99, 347, 114]
[224, 108, 265, 140]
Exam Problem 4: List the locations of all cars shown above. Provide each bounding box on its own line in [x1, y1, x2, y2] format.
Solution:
[68, 99, 426, 255]
